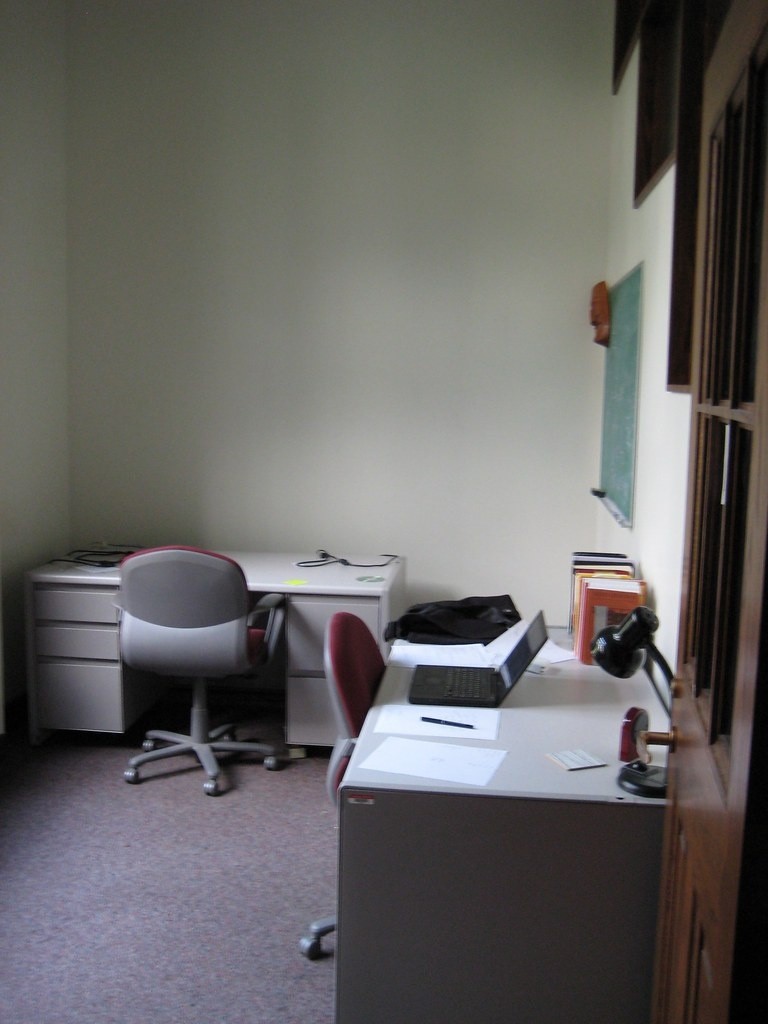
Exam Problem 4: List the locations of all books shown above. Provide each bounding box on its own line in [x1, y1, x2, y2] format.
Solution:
[569, 551, 647, 665]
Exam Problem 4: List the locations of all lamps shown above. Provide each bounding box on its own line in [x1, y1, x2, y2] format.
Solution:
[590, 606, 674, 797]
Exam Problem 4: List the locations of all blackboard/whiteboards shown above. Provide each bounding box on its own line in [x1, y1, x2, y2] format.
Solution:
[597, 260, 644, 528]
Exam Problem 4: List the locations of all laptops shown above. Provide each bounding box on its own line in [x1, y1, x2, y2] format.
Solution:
[409, 611, 549, 708]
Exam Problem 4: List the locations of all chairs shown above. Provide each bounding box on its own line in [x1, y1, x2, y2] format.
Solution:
[111, 546, 285, 796]
[298, 612, 385, 959]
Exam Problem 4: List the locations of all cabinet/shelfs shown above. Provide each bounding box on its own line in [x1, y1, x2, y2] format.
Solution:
[652, 1, 768, 1024]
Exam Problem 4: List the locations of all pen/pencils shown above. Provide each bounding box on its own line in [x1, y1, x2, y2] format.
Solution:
[421, 716, 473, 729]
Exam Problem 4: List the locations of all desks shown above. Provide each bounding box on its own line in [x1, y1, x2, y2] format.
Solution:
[335, 627, 673, 1024]
[24, 544, 405, 759]
